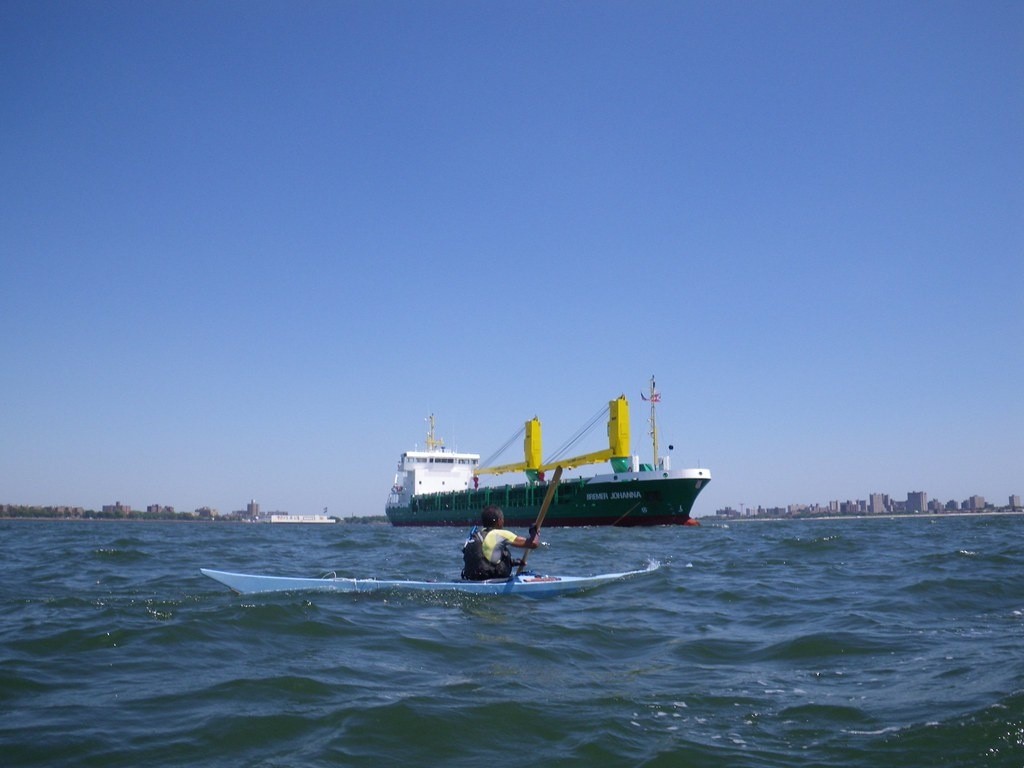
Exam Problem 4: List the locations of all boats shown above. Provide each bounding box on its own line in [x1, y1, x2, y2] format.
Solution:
[199, 566, 658, 597]
[386, 374, 714, 526]
[264, 514, 336, 524]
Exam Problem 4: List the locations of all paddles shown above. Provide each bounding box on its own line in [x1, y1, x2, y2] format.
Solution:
[514, 465, 562, 575]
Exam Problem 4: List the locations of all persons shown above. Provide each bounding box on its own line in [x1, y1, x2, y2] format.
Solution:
[462, 503, 540, 581]
